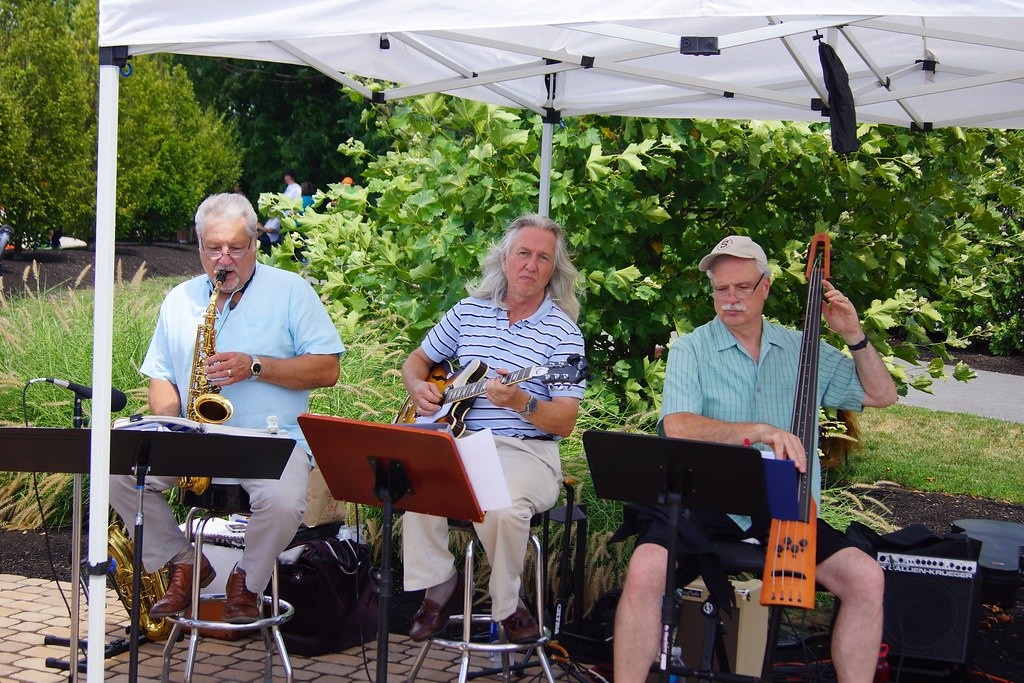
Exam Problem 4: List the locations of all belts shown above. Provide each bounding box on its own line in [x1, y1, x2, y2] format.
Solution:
[525, 435, 555, 441]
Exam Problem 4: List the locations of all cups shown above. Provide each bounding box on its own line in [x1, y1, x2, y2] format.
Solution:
[491, 640, 515, 676]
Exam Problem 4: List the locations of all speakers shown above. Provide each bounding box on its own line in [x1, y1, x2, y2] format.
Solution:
[877, 552, 984, 668]
[646, 578, 769, 683]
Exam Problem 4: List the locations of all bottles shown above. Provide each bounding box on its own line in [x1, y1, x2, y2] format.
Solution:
[668, 646, 685, 683]
[873, 643, 889, 683]
[488, 607, 508, 661]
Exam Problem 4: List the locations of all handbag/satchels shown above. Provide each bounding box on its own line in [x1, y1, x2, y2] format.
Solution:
[267, 536, 381, 657]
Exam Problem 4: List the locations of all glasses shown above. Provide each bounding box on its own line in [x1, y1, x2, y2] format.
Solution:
[708, 274, 764, 299]
[201, 236, 252, 260]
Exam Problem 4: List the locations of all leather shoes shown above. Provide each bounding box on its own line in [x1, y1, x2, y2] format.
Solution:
[409, 567, 465, 642]
[501, 599, 541, 644]
[222, 561, 259, 623]
[148, 553, 216, 618]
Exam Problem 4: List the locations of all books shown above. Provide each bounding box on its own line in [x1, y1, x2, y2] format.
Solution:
[113, 414, 290, 439]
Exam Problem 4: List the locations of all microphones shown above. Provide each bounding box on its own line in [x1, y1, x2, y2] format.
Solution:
[45, 378, 126, 412]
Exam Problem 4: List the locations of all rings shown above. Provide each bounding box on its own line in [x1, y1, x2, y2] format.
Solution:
[490, 392, 495, 397]
[416, 406, 422, 413]
[845, 297, 848, 303]
[228, 370, 232, 377]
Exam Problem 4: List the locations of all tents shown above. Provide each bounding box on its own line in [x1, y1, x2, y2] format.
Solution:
[87, 0, 1024, 683]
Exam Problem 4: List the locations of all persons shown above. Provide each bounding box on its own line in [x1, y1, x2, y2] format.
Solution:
[609, 236, 901, 683]
[109, 193, 344, 623]
[401, 214, 588, 645]
[330, 176, 354, 207]
[257, 172, 302, 255]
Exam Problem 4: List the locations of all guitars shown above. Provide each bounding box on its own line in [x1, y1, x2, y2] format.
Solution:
[393, 352, 598, 438]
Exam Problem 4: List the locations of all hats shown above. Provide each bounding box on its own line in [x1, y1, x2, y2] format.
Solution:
[341, 177, 354, 184]
[697, 235, 767, 270]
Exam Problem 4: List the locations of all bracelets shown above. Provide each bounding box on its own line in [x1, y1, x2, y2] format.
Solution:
[845, 332, 868, 350]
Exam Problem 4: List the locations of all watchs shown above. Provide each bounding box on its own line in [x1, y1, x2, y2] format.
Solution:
[519, 395, 538, 417]
[248, 354, 262, 381]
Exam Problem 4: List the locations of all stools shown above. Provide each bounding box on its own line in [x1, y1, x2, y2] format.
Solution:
[648, 534, 841, 683]
[406, 511, 555, 683]
[158, 483, 294, 683]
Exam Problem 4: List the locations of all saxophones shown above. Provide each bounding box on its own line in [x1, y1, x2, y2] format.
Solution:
[179, 268, 235, 497]
[107, 518, 174, 643]
